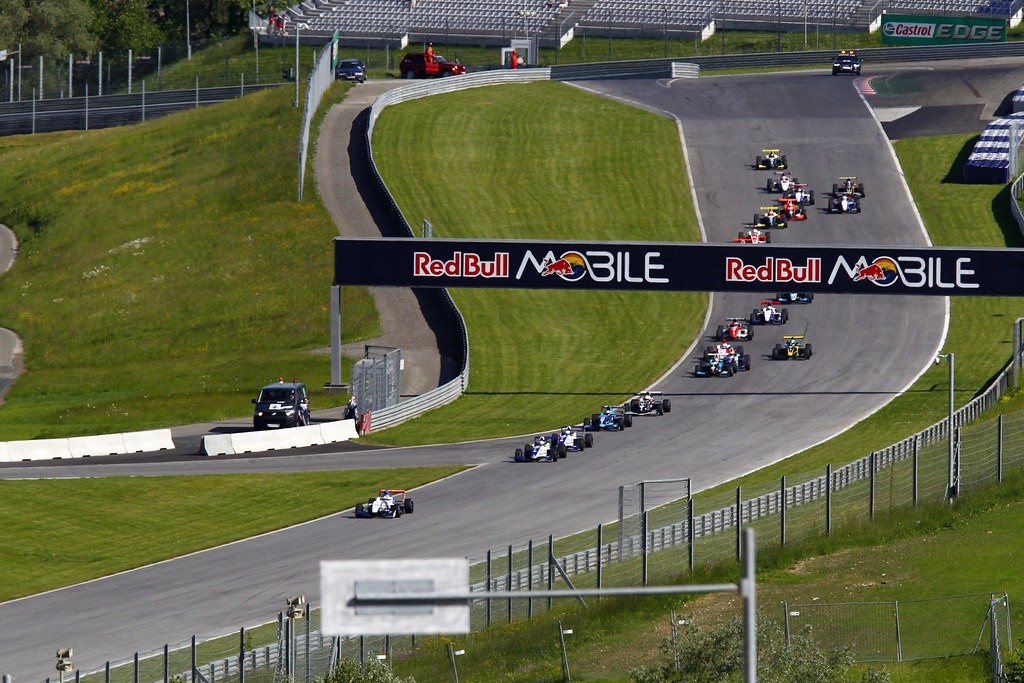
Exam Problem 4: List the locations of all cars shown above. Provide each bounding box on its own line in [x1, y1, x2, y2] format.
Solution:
[336, 58, 368, 84]
[832, 50, 863, 76]
[400, 52, 466, 80]
[250, 383, 309, 429]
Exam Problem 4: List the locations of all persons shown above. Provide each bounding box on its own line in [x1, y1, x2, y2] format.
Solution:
[383, 491, 389, 497]
[511, 50, 518, 69]
[566, 425, 571, 430]
[426, 43, 434, 64]
[539, 436, 545, 440]
[846, 178, 851, 186]
[345, 396, 358, 419]
[769, 151, 774, 157]
[644, 392, 650, 398]
[546, 0, 568, 10]
[268, 14, 286, 35]
[606, 406, 611, 412]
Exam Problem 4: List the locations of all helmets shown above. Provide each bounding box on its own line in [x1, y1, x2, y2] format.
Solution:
[605, 410, 611, 415]
[732, 321, 738, 326]
[383, 491, 388, 496]
[790, 340, 795, 343]
[539, 441, 545, 445]
[644, 395, 651, 400]
[722, 343, 728, 348]
[714, 355, 719, 361]
[566, 430, 570, 434]
